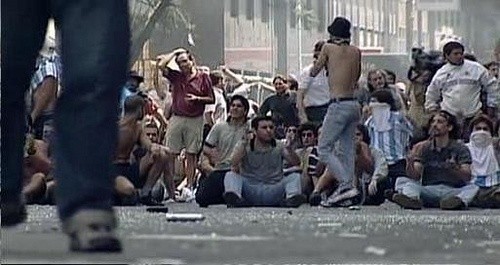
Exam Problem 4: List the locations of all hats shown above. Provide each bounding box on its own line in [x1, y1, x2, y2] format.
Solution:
[469, 115, 495, 138]
[328, 17, 351, 39]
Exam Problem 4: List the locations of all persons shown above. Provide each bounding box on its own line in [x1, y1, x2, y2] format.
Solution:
[0, 0, 130, 253]
[309, 17, 361, 207]
[20, 41, 500, 211]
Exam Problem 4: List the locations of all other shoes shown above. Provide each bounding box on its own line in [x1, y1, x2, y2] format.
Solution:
[321, 199, 353, 207]
[70, 221, 122, 252]
[0, 191, 28, 226]
[141, 190, 164, 206]
[392, 193, 423, 209]
[226, 192, 239, 207]
[439, 196, 462, 209]
[286, 194, 308, 208]
[327, 186, 359, 203]
[308, 193, 322, 207]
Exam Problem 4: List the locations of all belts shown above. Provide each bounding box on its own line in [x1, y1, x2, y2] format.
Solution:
[329, 97, 357, 104]
